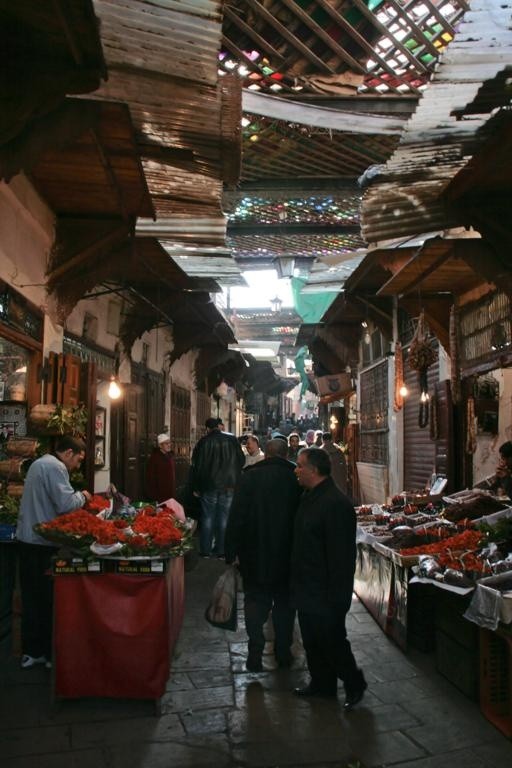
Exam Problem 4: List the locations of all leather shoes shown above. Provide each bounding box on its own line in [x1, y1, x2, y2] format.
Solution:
[344, 681, 367, 708]
[294, 686, 315, 695]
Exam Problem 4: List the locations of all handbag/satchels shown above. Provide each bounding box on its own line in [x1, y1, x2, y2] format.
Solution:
[204, 567, 238, 631]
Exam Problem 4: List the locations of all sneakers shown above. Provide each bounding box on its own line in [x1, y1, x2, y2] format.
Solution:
[20, 653, 53, 670]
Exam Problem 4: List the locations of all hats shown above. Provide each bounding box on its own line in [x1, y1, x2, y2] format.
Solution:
[158, 433, 169, 445]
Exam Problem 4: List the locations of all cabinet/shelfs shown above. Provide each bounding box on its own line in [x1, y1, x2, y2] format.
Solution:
[95, 405, 106, 468]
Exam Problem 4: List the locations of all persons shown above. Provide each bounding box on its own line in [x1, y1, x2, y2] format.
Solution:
[15, 435, 91, 670]
[292, 443, 365, 712]
[492, 440, 511, 504]
[145, 434, 176, 505]
[227, 432, 304, 671]
[191, 416, 347, 561]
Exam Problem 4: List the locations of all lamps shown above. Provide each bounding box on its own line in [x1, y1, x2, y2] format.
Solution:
[272, 248, 298, 279]
[270, 296, 282, 313]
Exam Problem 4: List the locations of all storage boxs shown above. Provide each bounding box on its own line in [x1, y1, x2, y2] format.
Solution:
[314, 372, 352, 396]
